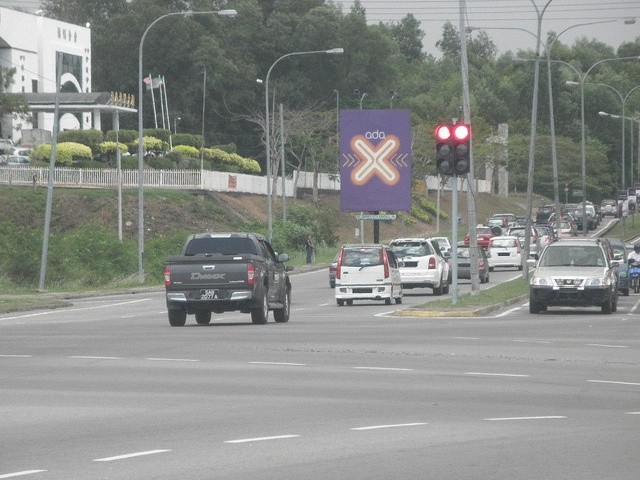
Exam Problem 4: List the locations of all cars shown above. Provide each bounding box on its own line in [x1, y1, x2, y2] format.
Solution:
[463, 224, 494, 249]
[4, 155, 34, 168]
[432, 237, 451, 258]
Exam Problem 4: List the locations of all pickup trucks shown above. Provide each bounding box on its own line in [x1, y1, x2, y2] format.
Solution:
[165, 232, 294, 326]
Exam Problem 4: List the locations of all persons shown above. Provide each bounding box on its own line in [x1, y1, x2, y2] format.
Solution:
[628, 243, 640, 290]
[305, 233, 315, 264]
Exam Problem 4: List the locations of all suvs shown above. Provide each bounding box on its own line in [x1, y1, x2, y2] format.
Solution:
[507, 226, 541, 260]
[487, 236, 523, 270]
[529, 241, 619, 314]
[609, 240, 629, 296]
[389, 238, 449, 295]
[494, 213, 517, 226]
[600, 199, 622, 217]
[335, 244, 402, 303]
[11, 147, 36, 156]
[536, 206, 556, 226]
[445, 245, 489, 283]
[486, 217, 511, 235]
[551, 220, 578, 239]
[574, 205, 600, 229]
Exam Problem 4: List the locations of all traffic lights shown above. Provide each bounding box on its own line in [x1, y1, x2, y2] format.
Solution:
[454, 123, 470, 175]
[435, 123, 454, 176]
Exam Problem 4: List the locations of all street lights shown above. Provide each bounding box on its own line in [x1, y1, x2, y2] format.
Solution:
[266, 48, 345, 244]
[566, 81, 640, 190]
[511, 56, 640, 239]
[138, 9, 238, 286]
[599, 111, 640, 187]
[256, 78, 275, 176]
[465, 18, 636, 241]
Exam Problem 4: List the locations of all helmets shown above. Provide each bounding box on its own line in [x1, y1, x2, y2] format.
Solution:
[634, 244, 640, 252]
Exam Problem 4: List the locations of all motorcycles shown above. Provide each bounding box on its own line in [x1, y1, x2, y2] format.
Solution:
[629, 260, 640, 293]
